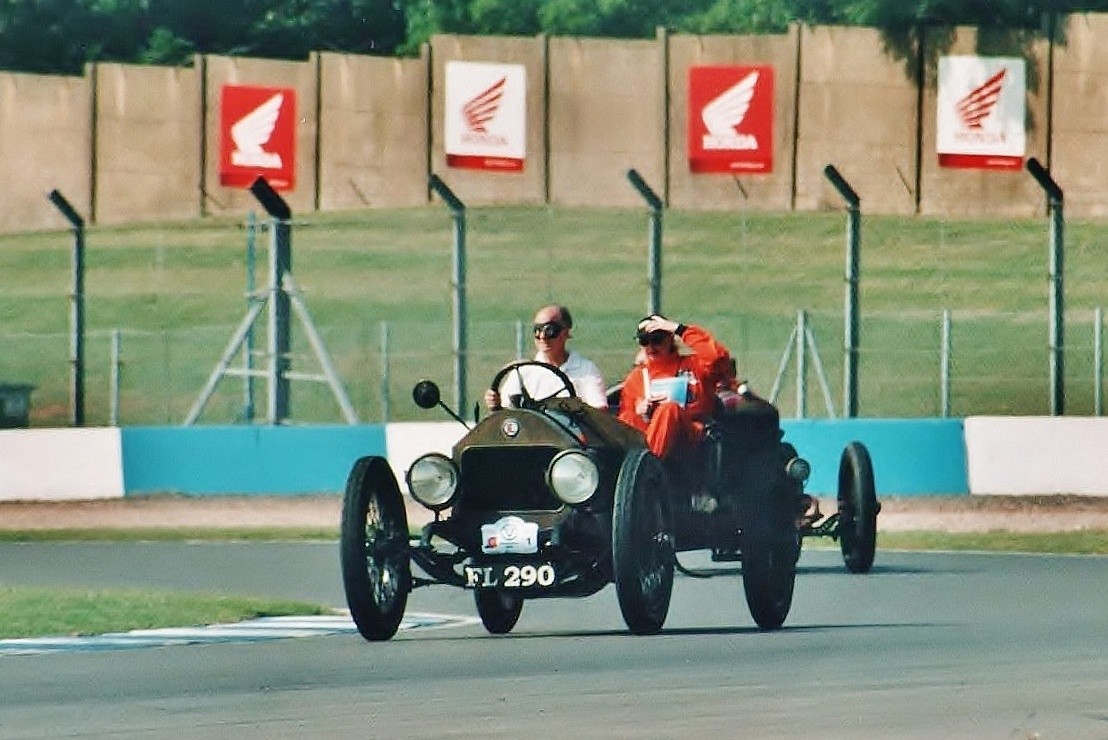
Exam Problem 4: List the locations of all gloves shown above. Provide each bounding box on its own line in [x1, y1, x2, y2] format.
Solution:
[638, 314, 679, 334]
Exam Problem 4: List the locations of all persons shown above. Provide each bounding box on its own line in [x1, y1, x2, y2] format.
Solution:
[716, 358, 737, 393]
[619, 313, 729, 457]
[486, 302, 608, 444]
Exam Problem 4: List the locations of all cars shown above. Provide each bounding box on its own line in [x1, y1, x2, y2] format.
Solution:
[338, 359, 809, 640]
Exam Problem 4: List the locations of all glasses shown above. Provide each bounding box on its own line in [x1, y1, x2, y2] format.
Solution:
[638, 335, 665, 346]
[533, 324, 565, 339]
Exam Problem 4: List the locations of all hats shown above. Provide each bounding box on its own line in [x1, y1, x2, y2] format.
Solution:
[633, 313, 672, 338]
